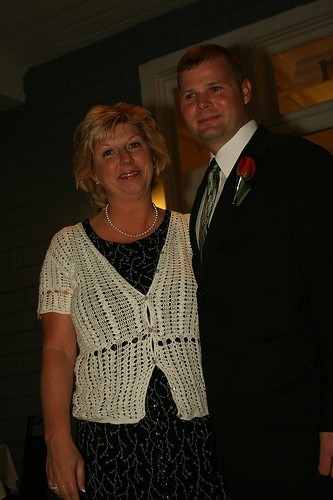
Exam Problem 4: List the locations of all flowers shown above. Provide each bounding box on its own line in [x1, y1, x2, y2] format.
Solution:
[235, 156, 256, 207]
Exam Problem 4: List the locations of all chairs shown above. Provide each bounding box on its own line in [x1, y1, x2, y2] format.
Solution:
[20, 415, 48, 500]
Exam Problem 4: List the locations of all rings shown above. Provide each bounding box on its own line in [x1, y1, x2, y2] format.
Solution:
[50, 486, 58, 490]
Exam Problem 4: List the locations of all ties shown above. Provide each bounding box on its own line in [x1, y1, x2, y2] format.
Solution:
[198, 162, 221, 264]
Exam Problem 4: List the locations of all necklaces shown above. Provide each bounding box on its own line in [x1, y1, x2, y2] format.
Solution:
[105, 203, 158, 237]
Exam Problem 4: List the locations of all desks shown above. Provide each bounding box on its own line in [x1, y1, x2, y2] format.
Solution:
[0, 444, 20, 500]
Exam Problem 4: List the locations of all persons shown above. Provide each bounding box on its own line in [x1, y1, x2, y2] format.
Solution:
[37, 102, 235, 500]
[176, 44, 333, 500]
